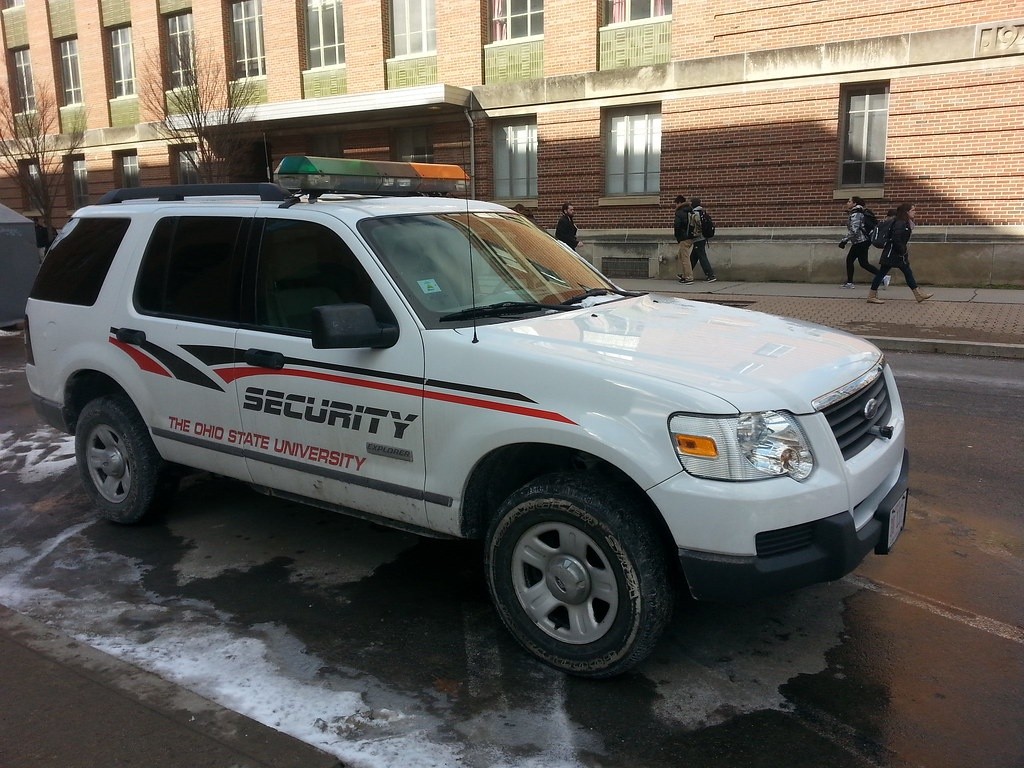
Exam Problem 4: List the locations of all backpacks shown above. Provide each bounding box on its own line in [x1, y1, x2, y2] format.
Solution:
[870, 215, 897, 249]
[686, 211, 703, 238]
[850, 208, 877, 239]
[695, 210, 715, 239]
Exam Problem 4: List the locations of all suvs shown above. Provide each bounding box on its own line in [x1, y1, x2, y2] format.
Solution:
[20, 150, 913, 686]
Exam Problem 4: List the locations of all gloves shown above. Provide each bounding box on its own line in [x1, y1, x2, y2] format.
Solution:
[839, 241, 847, 249]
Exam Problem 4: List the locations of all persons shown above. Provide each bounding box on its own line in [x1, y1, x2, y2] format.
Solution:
[555, 203, 584, 252]
[32, 218, 58, 265]
[674, 195, 716, 285]
[514, 203, 538, 224]
[839, 196, 891, 291]
[867, 203, 934, 304]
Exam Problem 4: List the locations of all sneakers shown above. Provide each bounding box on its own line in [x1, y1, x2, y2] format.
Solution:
[839, 282, 855, 289]
[702, 273, 717, 283]
[676, 273, 683, 279]
[882, 275, 891, 290]
[676, 279, 693, 285]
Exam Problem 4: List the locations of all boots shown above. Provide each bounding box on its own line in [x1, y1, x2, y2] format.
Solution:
[866, 289, 885, 304]
[912, 287, 935, 303]
